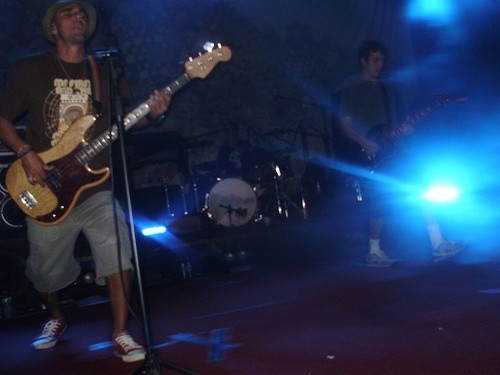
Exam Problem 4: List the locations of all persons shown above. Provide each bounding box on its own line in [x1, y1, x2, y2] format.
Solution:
[0, 0, 149, 361]
[334, 40, 467, 267]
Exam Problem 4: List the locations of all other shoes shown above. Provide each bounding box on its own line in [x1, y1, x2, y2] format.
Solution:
[367, 250, 400, 268]
[431, 241, 464, 257]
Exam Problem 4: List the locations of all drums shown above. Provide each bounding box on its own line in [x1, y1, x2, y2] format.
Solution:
[271, 155, 294, 182]
[205, 176, 263, 227]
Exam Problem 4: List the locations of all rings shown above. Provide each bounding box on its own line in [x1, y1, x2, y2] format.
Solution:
[28, 176, 37, 181]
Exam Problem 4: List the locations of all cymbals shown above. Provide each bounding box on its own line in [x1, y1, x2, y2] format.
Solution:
[194, 160, 236, 170]
[259, 127, 296, 137]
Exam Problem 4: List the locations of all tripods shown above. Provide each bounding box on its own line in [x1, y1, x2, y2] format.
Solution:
[109, 57, 200, 375]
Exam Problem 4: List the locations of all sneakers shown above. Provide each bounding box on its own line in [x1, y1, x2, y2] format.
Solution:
[112, 334, 146, 363]
[32, 314, 67, 350]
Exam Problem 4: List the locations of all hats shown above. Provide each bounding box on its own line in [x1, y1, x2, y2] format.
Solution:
[42, 0, 97, 45]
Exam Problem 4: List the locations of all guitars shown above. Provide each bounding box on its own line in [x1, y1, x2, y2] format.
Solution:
[4, 42, 232, 225]
[351, 87, 467, 174]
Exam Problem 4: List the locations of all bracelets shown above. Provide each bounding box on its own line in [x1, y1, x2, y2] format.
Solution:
[145, 111, 166, 128]
[17, 143, 34, 158]
